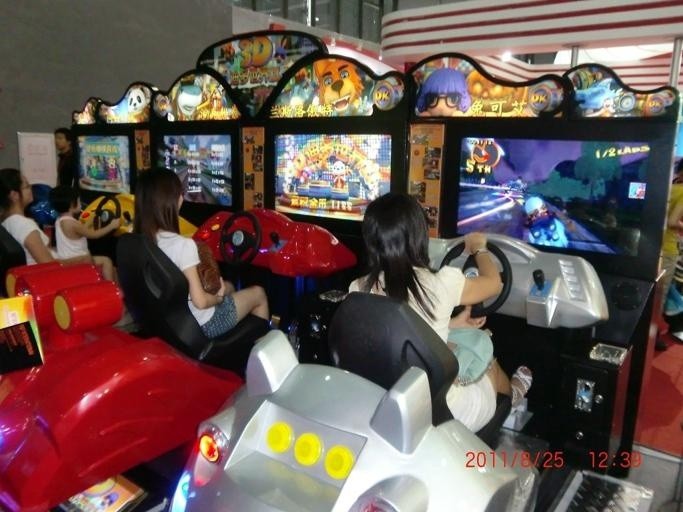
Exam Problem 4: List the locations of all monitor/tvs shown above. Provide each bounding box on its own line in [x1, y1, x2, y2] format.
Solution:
[73, 134, 133, 196]
[158, 134, 235, 209]
[454, 131, 655, 261]
[266, 130, 395, 225]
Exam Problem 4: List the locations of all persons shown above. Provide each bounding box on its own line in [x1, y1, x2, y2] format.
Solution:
[349, 192, 535, 433]
[655, 157, 683, 352]
[0, 168, 119, 283]
[54, 127, 77, 186]
[128, 167, 273, 339]
[49, 184, 121, 282]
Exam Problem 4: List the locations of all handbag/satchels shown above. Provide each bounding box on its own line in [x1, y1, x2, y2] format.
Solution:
[445, 326, 496, 387]
[197, 242, 221, 295]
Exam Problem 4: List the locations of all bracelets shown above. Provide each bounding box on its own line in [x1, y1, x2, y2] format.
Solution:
[472, 247, 489, 257]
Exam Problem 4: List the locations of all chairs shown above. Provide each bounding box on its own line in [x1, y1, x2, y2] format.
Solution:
[332, 294, 512, 457]
[118, 233, 268, 365]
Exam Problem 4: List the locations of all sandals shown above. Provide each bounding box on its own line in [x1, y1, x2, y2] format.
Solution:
[510, 366, 533, 406]
